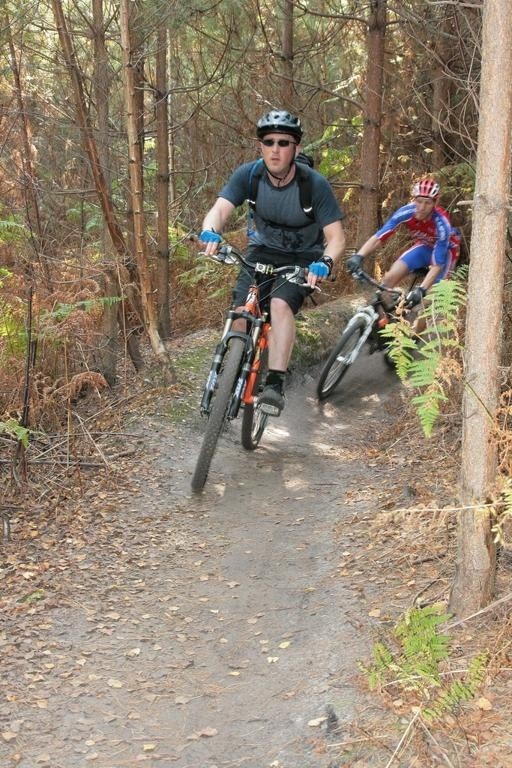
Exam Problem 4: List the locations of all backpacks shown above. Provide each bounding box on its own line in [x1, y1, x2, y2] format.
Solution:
[249, 153, 315, 230]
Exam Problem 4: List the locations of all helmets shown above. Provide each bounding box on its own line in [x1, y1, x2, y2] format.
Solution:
[255, 110, 303, 142]
[413, 179, 441, 200]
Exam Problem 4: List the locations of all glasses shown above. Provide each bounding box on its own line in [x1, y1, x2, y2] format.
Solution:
[261, 139, 298, 147]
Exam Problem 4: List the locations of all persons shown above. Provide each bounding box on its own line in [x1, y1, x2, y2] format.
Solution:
[198, 111, 346, 417]
[345, 179, 461, 353]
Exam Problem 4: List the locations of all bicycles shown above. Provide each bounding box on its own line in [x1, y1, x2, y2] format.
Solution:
[190, 235, 336, 488]
[318, 268, 426, 399]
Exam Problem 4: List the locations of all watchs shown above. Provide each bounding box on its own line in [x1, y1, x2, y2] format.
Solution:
[318, 256, 333, 269]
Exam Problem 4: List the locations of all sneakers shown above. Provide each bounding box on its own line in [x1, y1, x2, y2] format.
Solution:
[260, 386, 285, 418]
